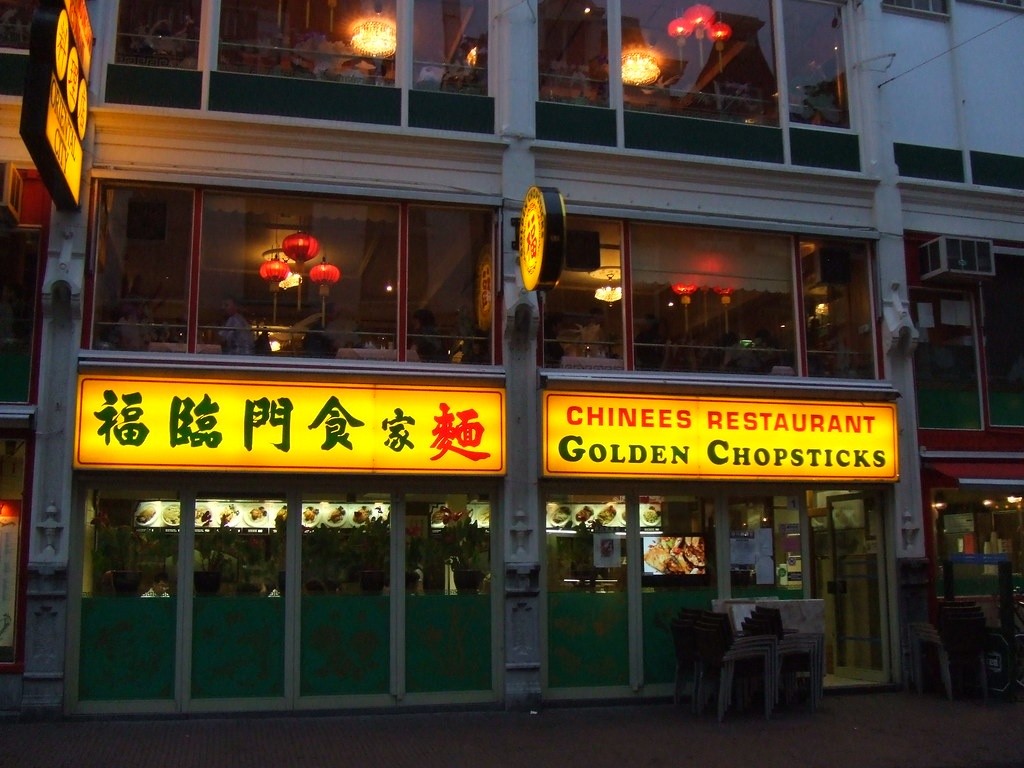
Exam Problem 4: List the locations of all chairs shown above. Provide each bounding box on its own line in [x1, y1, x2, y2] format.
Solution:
[907, 601, 991, 707]
[669, 605, 825, 724]
[443, 339, 474, 364]
[250, 326, 273, 355]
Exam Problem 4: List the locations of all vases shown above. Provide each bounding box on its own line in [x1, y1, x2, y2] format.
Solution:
[453, 570, 486, 594]
[278, 571, 305, 594]
[110, 569, 144, 595]
[194, 571, 222, 595]
[358, 570, 385, 594]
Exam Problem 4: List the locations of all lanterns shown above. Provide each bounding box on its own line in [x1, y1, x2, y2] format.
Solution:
[668, 4, 733, 73]
[671, 250, 738, 335]
[258, 232, 341, 329]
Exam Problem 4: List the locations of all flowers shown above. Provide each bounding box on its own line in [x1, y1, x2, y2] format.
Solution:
[194, 512, 241, 571]
[90, 512, 177, 581]
[347, 512, 391, 587]
[274, 514, 306, 570]
[446, 518, 491, 583]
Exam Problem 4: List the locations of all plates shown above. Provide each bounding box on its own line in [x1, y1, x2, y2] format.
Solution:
[136, 504, 371, 529]
[550, 506, 661, 526]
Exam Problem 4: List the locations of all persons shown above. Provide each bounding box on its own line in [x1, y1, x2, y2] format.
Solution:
[589, 307, 663, 366]
[409, 309, 443, 359]
[212, 295, 255, 356]
[308, 571, 420, 595]
[321, 303, 361, 348]
[543, 311, 565, 359]
[720, 318, 873, 380]
[117, 297, 170, 349]
[141, 573, 170, 598]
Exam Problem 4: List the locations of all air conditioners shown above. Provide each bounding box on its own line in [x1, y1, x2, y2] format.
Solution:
[918, 235, 996, 287]
[0, 160, 24, 226]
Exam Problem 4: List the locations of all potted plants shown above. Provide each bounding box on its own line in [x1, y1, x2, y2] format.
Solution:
[306, 523, 346, 593]
[384, 536, 420, 592]
[556, 521, 616, 593]
[236, 541, 269, 592]
[417, 534, 448, 594]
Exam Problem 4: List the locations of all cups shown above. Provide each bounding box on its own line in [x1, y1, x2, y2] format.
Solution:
[984, 531, 998, 554]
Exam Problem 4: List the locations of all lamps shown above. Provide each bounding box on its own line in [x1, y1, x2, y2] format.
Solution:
[621, 53, 661, 86]
[350, 21, 397, 57]
[594, 274, 622, 307]
[279, 259, 303, 290]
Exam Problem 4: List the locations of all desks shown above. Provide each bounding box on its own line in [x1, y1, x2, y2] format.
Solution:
[148, 342, 223, 354]
[334, 348, 421, 362]
[78, 589, 804, 700]
